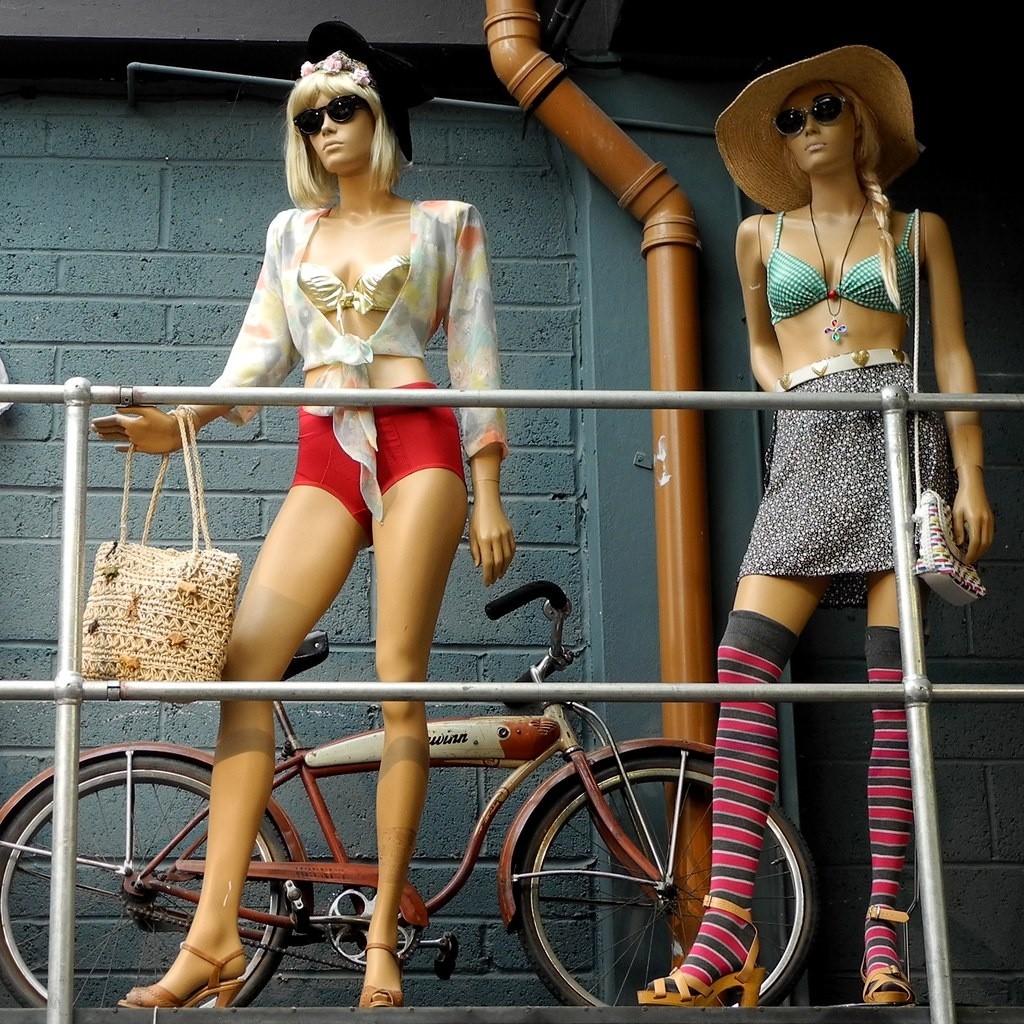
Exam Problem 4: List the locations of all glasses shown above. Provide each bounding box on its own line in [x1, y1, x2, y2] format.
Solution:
[771, 94, 851, 135]
[293, 92, 372, 134]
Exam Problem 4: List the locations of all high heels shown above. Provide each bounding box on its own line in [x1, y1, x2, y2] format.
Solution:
[860, 904, 917, 1003]
[637, 895, 766, 1007]
[117, 941, 247, 1009]
[359, 943, 403, 1006]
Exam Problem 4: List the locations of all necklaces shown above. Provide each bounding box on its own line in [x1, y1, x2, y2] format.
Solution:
[808, 196, 869, 342]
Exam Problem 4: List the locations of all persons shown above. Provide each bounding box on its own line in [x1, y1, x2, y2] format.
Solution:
[638, 42, 993, 1001]
[89, 18, 518, 1007]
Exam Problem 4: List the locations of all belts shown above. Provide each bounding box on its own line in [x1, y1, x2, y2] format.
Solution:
[777, 347, 913, 392]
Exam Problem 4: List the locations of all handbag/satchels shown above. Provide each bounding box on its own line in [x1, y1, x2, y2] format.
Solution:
[913, 488, 987, 607]
[80, 404, 242, 682]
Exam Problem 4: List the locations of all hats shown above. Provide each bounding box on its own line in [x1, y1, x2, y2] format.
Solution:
[714, 45, 919, 214]
[308, 20, 435, 161]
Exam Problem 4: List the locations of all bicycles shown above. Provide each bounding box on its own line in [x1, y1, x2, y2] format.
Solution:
[1, 578, 824, 1008]
